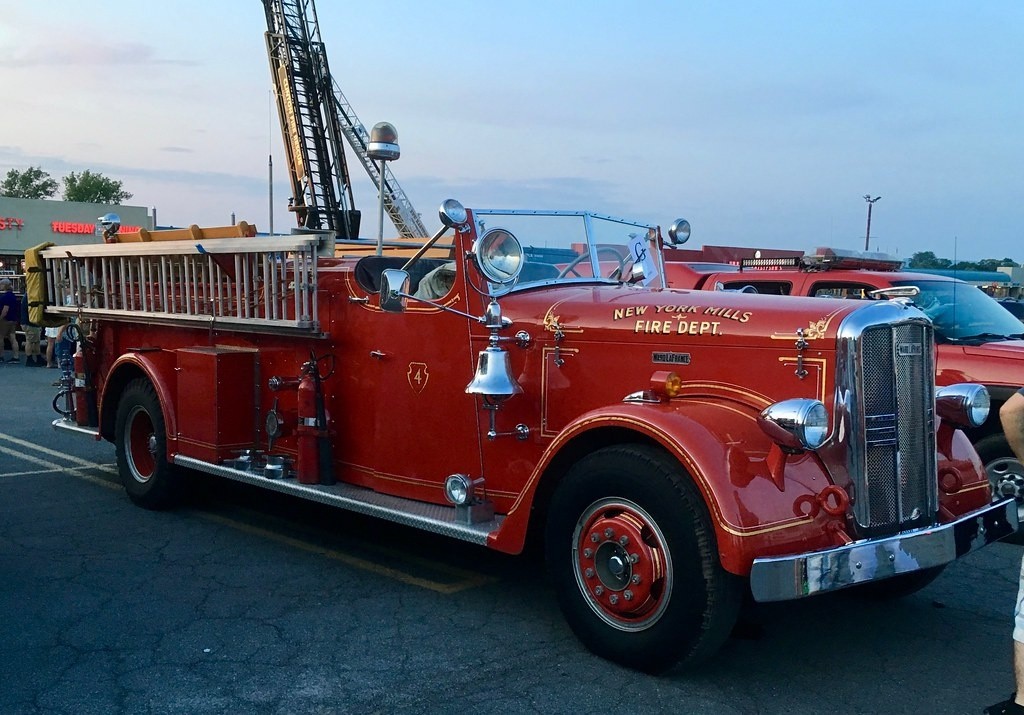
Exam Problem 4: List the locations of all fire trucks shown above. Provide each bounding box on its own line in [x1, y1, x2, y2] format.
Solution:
[25, 1, 1013, 665]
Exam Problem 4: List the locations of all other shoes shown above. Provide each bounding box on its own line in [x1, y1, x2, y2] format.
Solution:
[25, 355, 36, 366]
[46, 362, 58, 368]
[35, 355, 47, 367]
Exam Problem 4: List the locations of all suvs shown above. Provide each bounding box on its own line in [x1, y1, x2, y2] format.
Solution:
[698, 252, 1024, 481]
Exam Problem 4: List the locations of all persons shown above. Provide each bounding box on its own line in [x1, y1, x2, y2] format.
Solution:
[0, 279, 19, 364]
[45, 283, 101, 368]
[20, 290, 48, 366]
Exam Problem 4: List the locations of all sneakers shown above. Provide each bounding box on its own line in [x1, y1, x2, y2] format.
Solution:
[0, 357, 5, 363]
[7, 357, 20, 364]
